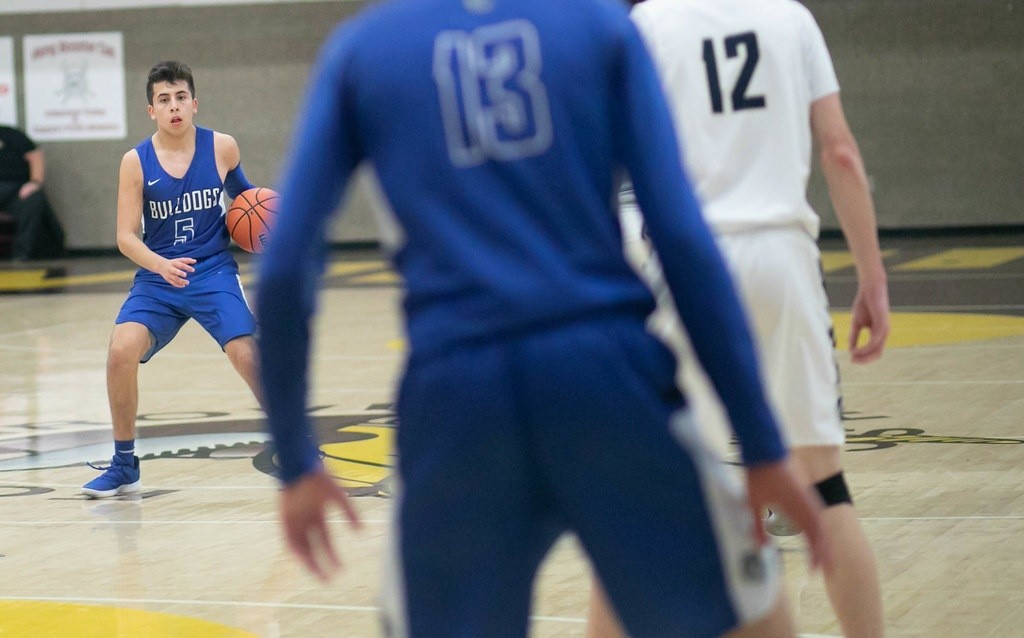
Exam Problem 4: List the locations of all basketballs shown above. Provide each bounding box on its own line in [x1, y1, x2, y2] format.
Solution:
[228, 188, 280, 253]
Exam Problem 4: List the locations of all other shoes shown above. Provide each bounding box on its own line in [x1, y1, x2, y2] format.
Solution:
[766, 512, 802, 537]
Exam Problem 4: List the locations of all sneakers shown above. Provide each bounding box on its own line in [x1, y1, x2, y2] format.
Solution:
[82, 455, 141, 497]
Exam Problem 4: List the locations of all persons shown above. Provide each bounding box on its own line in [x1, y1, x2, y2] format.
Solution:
[82, 60, 262, 498]
[253, 0, 890, 638]
[0, 125, 67, 293]
